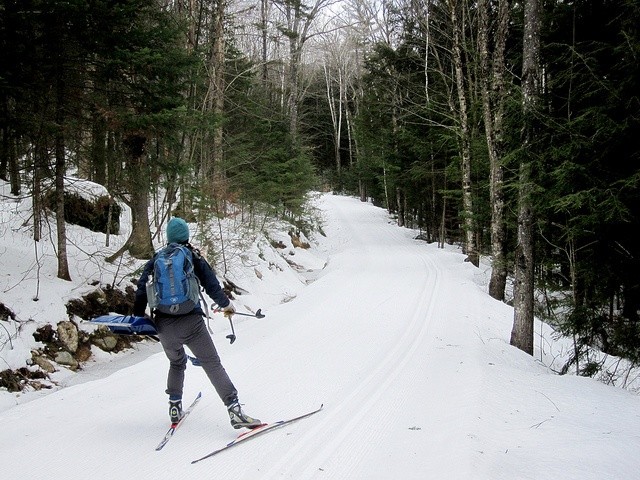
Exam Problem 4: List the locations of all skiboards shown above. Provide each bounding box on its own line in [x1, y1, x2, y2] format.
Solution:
[155, 392, 323, 464]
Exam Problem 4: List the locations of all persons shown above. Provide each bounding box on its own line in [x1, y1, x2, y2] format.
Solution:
[133, 217, 262, 428]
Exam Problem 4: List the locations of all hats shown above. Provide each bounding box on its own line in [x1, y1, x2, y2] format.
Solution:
[166, 218, 190, 244]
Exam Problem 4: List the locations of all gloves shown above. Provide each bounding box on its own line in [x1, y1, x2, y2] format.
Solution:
[224, 301, 236, 317]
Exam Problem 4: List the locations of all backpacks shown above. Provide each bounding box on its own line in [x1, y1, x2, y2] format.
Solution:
[145, 242, 214, 337]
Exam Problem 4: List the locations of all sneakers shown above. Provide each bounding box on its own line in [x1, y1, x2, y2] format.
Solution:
[168, 397, 185, 424]
[228, 401, 261, 428]
